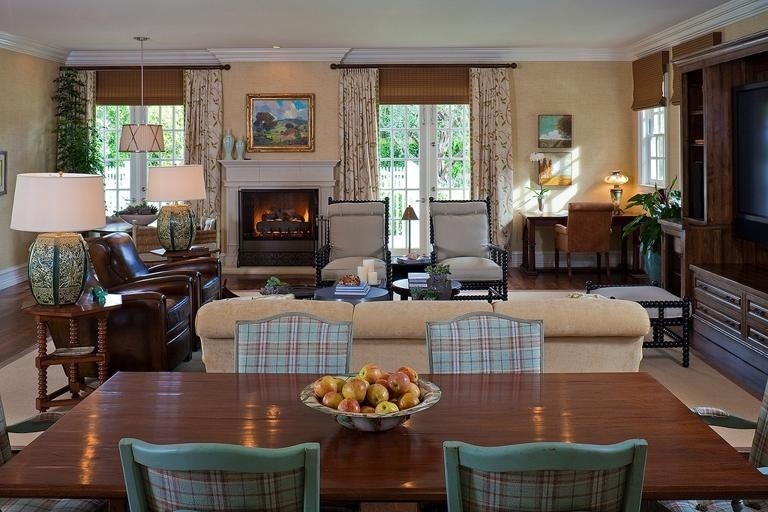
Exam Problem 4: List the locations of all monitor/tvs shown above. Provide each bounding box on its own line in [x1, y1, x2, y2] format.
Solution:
[732, 81, 768, 224]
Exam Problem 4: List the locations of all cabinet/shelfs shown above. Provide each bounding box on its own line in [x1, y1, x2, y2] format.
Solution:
[673, 61, 733, 227]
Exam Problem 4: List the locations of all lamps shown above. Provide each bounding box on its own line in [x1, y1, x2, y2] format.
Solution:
[118, 35, 165, 155]
[9, 173, 106, 305]
[605, 170, 628, 214]
[147, 163, 207, 250]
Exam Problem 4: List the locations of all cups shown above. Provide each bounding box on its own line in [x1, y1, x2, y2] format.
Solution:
[358, 257, 377, 284]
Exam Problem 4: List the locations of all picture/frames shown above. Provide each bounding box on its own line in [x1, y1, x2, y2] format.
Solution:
[0, 151, 8, 195]
[246, 93, 316, 154]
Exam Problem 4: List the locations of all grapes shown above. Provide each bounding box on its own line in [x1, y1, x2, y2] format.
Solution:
[260, 284, 292, 294]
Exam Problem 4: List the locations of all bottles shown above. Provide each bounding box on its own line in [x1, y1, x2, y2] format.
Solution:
[89, 285, 104, 297]
[222, 128, 247, 161]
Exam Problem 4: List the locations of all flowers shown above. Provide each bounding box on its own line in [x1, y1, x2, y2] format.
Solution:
[523, 151, 550, 199]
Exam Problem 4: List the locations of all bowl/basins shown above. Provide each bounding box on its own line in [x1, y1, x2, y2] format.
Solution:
[119, 214, 158, 226]
[299, 374, 441, 431]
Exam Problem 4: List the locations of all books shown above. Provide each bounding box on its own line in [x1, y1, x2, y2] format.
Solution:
[334, 280, 370, 296]
[407, 272, 430, 288]
[397, 257, 431, 264]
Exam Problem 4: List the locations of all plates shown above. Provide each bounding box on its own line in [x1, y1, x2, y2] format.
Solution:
[54, 347, 94, 357]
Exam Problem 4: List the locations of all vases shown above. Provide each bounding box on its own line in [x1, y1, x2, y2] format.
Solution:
[224, 128, 245, 161]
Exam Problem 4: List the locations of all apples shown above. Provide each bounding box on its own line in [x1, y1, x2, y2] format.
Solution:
[313, 363, 421, 414]
